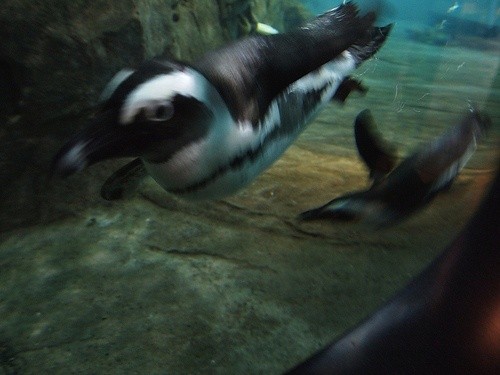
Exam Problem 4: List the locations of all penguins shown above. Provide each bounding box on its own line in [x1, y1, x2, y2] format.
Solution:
[55, 1, 394, 203]
[297, 108, 495, 233]
